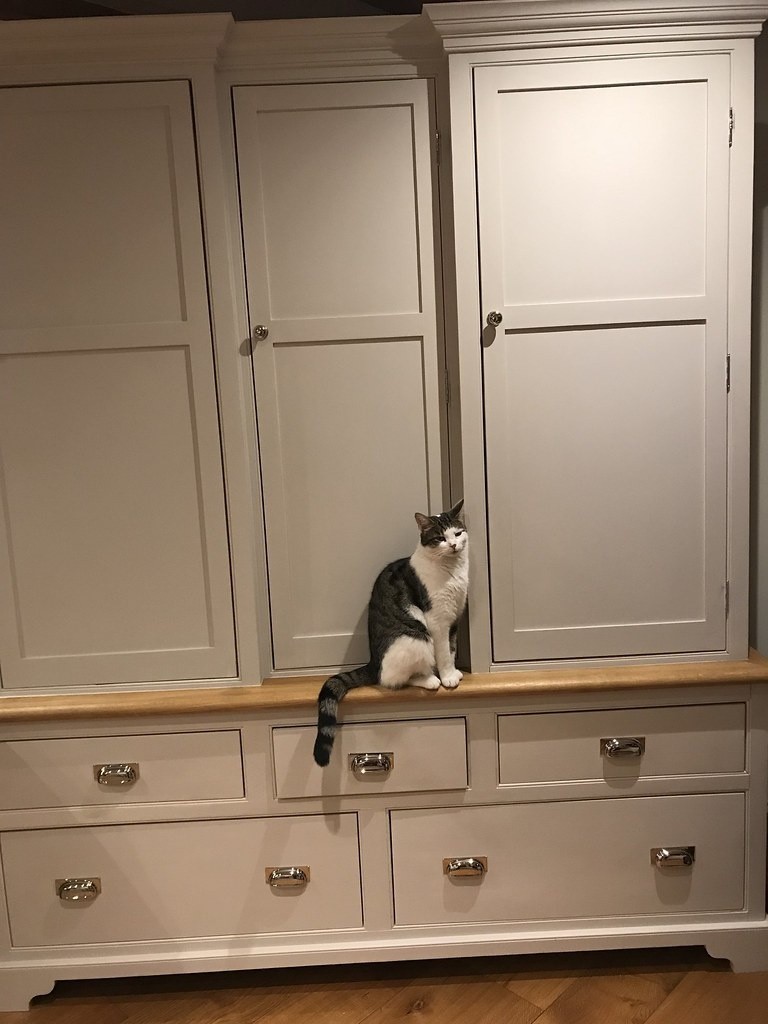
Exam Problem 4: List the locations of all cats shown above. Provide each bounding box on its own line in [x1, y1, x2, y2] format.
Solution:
[312, 498, 470, 767]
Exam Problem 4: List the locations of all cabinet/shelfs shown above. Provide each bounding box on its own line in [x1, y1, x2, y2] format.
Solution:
[0, 652, 768, 1014]
[0, 0, 768, 699]
